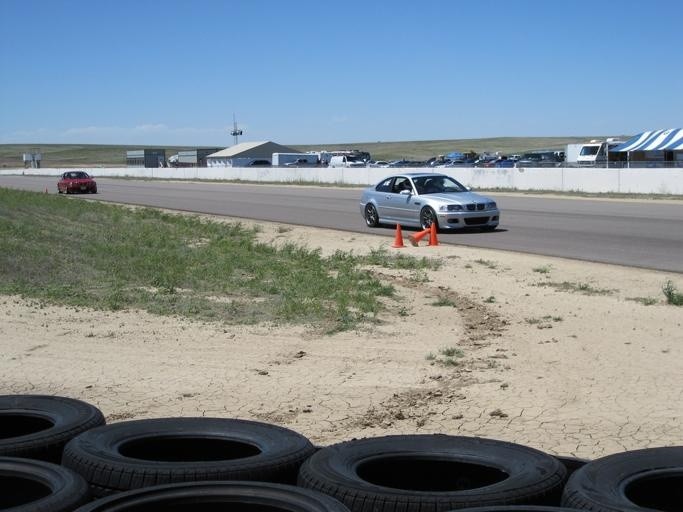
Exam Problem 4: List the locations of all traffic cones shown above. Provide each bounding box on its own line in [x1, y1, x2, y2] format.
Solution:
[391, 223, 408, 248]
[407, 227, 431, 247]
[428, 223, 438, 245]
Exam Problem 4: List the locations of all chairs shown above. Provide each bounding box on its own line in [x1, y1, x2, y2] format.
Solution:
[399, 180, 433, 194]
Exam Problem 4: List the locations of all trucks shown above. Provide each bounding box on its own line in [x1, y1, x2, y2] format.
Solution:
[271, 151, 332, 168]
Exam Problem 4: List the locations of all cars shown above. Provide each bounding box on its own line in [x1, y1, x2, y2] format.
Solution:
[57, 172, 97, 194]
[359, 172, 500, 231]
